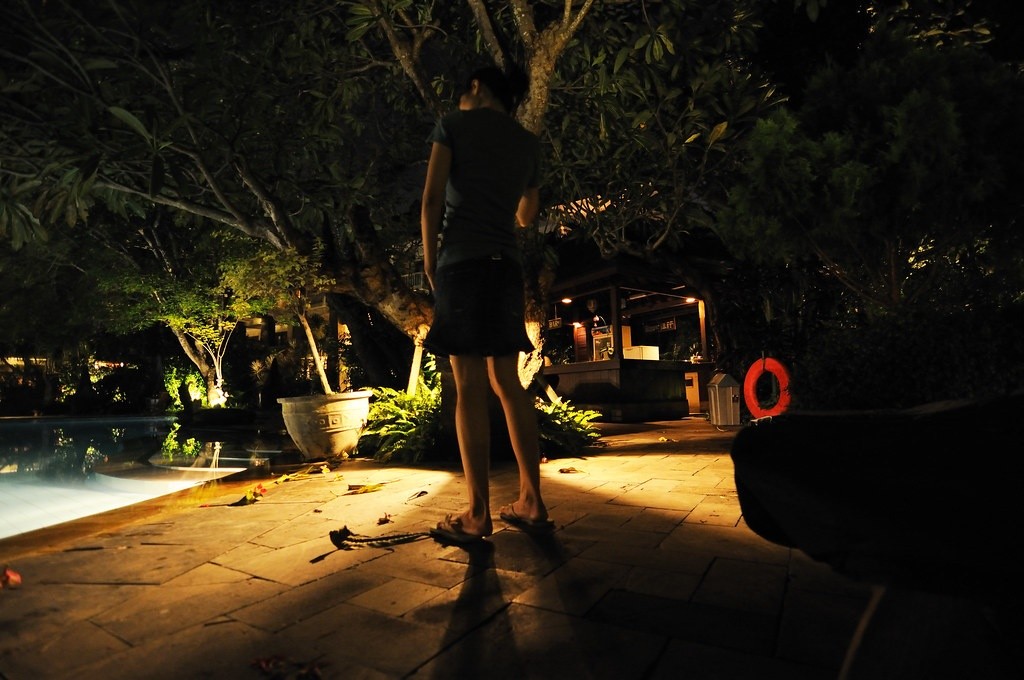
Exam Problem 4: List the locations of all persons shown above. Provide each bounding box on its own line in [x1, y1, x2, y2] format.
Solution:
[420, 62, 554, 542]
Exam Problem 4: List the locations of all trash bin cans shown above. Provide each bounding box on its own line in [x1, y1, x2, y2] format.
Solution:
[685, 371, 701, 415]
[706, 372, 744, 426]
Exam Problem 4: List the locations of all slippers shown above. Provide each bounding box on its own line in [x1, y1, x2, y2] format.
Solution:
[430, 514, 481, 542]
[500, 504, 554, 527]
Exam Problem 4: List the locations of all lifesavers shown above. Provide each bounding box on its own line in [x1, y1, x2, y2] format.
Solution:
[743, 357, 792, 419]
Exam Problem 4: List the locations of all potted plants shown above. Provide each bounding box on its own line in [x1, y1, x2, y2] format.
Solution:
[210, 225, 374, 461]
